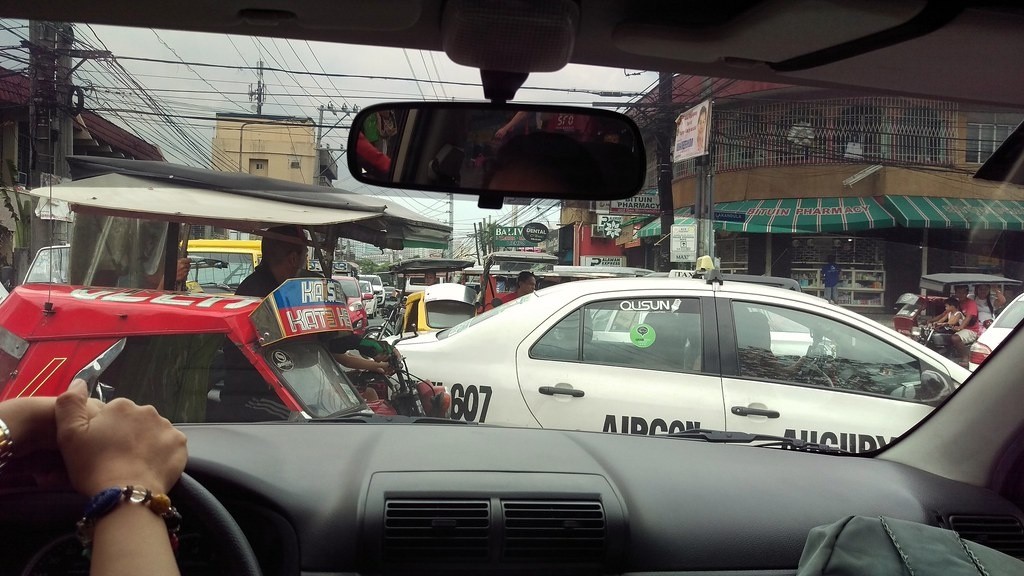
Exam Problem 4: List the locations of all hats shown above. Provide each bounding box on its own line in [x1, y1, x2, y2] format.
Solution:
[261, 226, 314, 264]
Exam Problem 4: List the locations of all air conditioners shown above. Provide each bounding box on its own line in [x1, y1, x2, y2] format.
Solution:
[590, 223, 606, 238]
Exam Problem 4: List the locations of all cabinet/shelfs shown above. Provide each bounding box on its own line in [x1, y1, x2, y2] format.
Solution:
[790, 268, 886, 308]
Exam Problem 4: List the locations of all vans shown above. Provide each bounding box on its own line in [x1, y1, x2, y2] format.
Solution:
[174, 238, 311, 298]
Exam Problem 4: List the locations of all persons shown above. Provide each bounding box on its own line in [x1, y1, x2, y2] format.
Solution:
[425, 271, 437, 285]
[126, 221, 191, 290]
[0, 379, 189, 576]
[741, 313, 812, 379]
[695, 106, 707, 153]
[357, 110, 606, 198]
[926, 285, 1006, 366]
[822, 255, 840, 304]
[485, 272, 538, 312]
[220, 226, 402, 422]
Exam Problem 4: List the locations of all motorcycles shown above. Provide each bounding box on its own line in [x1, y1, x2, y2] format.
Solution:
[358, 259, 476, 361]
[402, 252, 559, 333]
[893, 273, 1022, 368]
[0, 159, 462, 427]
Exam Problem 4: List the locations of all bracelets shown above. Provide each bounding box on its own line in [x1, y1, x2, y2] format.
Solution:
[74, 484, 183, 557]
[0, 420, 14, 471]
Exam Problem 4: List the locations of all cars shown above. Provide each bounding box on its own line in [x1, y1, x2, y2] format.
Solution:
[331, 270, 447, 336]
[381, 272, 973, 454]
[969, 292, 1024, 374]
[20, 245, 73, 285]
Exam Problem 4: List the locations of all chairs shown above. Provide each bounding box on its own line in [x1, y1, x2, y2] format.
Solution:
[643, 312, 684, 369]
[556, 310, 594, 343]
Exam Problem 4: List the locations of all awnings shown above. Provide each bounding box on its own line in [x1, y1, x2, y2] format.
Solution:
[636, 196, 1024, 238]
